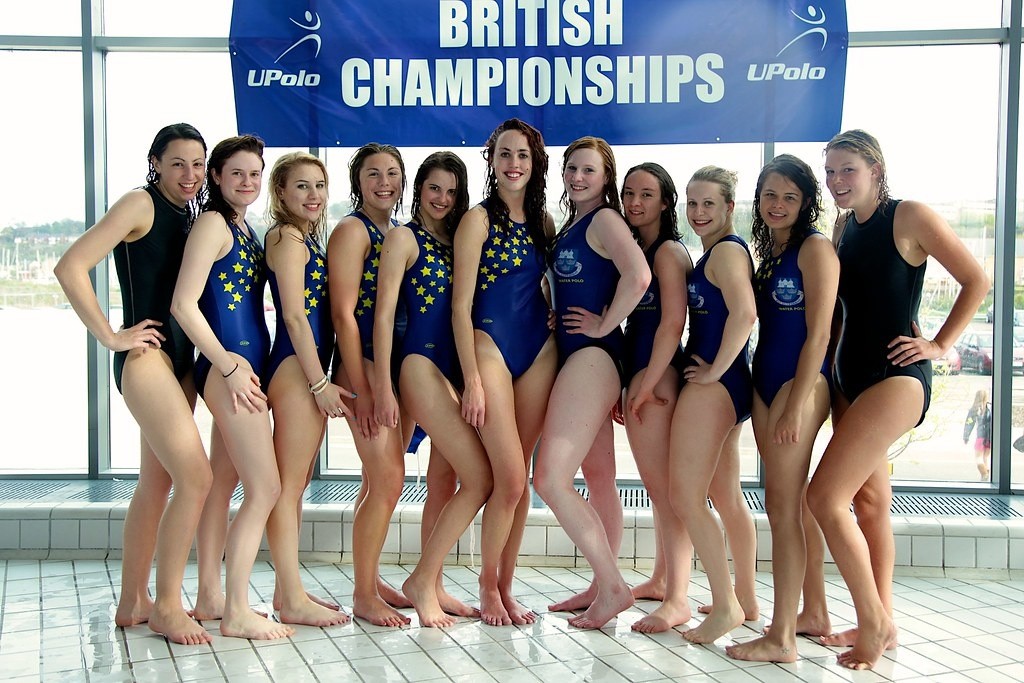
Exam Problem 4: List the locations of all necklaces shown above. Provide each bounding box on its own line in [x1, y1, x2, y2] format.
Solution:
[695, 248, 710, 264]
[361, 206, 376, 224]
[148, 185, 188, 215]
[585, 200, 603, 213]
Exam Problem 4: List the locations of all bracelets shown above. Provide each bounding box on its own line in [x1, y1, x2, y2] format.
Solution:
[223, 363, 239, 378]
[308, 376, 329, 396]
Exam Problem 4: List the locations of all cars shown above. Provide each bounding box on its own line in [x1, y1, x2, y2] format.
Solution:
[954, 330, 1024, 374]
[986, 304, 1020, 326]
[931, 347, 962, 376]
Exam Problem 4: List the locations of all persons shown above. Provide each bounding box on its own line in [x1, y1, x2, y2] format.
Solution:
[53, 121, 991, 672]
